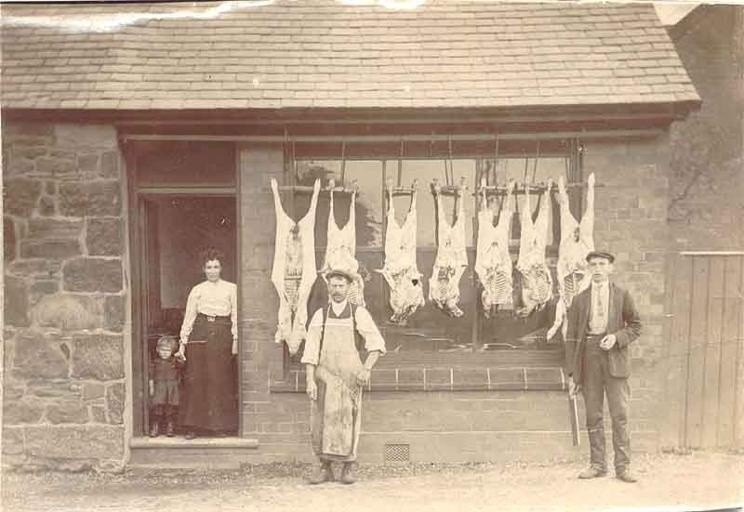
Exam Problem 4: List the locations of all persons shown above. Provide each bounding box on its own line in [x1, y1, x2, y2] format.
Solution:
[300, 268, 388, 484]
[147, 337, 184, 436]
[565, 249, 643, 484]
[174, 248, 239, 441]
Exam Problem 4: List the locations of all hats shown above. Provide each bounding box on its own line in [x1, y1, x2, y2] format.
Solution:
[325, 268, 352, 282]
[585, 250, 614, 263]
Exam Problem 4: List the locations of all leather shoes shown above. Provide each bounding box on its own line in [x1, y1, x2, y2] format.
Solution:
[614, 467, 637, 483]
[309, 466, 335, 486]
[577, 465, 609, 480]
[340, 464, 357, 485]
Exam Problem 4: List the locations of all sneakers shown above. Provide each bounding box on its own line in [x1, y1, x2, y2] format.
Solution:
[146, 425, 230, 440]
